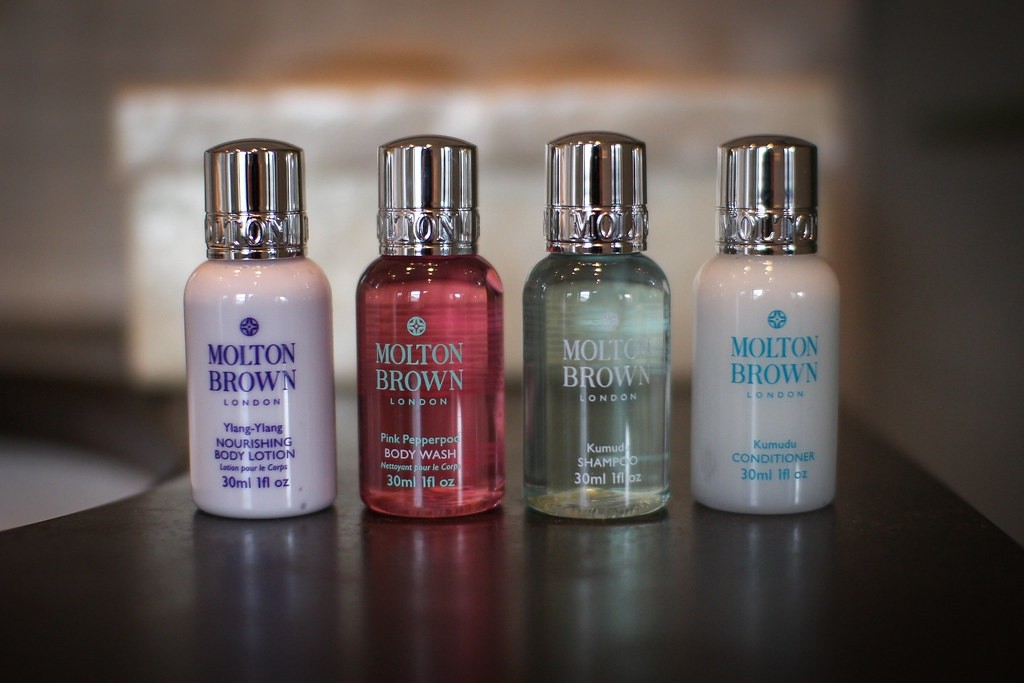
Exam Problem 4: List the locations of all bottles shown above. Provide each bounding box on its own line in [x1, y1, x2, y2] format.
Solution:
[524, 512, 672, 672]
[358, 506, 506, 663]
[696, 505, 836, 668]
[357, 136, 504, 519]
[195, 510, 337, 662]
[691, 133, 838, 514]
[183, 138, 339, 519]
[522, 132, 672, 518]
